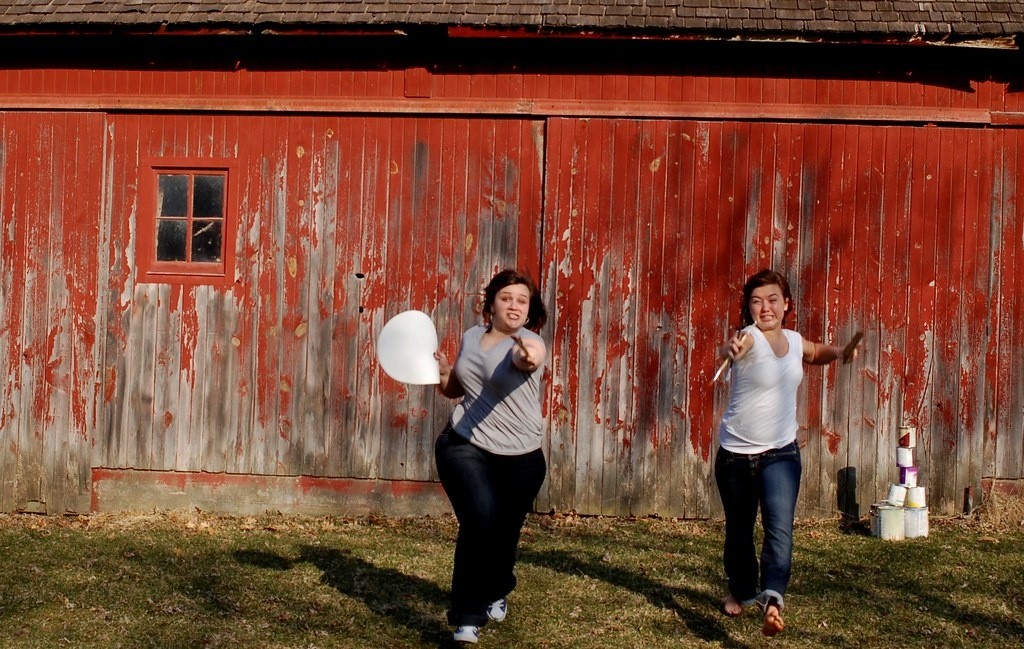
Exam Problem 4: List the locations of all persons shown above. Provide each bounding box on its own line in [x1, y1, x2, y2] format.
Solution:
[714, 267, 857, 637]
[433, 267, 547, 642]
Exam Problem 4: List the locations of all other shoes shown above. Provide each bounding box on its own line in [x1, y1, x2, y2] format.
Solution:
[486, 597, 508, 622]
[453, 625, 478, 644]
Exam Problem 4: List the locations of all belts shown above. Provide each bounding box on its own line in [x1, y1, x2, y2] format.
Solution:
[731, 446, 797, 460]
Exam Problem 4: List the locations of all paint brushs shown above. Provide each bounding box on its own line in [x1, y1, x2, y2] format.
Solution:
[510, 335, 530, 356]
[708, 313, 758, 387]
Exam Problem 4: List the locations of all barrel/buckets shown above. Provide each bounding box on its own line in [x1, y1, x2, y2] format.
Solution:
[877, 506, 905, 542]
[900, 467, 917, 487]
[904, 508, 928, 538]
[869, 503, 887, 536]
[907, 487, 926, 508]
[888, 485, 907, 506]
[898, 428, 916, 447]
[896, 447, 913, 467]
